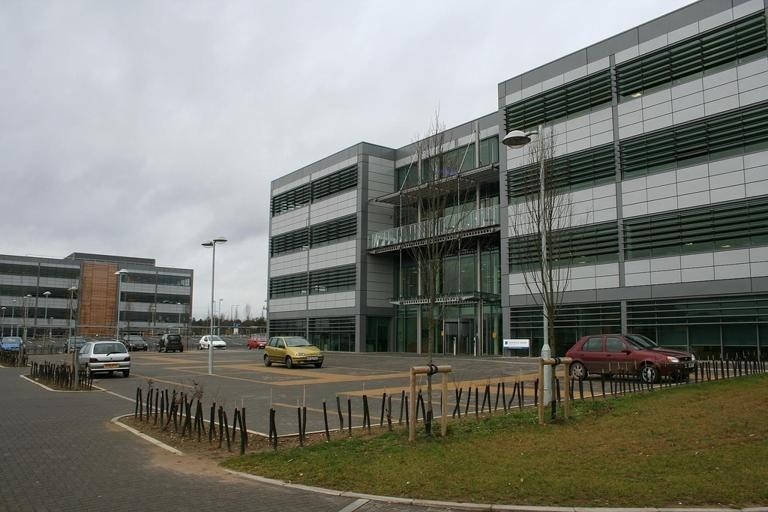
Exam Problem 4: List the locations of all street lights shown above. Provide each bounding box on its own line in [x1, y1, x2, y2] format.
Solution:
[1, 294, 32, 337]
[213, 299, 224, 336]
[67, 286, 78, 337]
[200, 238, 228, 342]
[43, 291, 52, 337]
[113, 268, 130, 339]
[499, 125, 551, 346]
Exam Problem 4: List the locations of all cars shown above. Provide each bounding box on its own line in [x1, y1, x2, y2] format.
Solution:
[0, 336, 24, 357]
[565, 333, 698, 384]
[122, 334, 148, 352]
[77, 340, 131, 379]
[196, 334, 227, 350]
[63, 336, 86, 353]
[156, 333, 184, 352]
[263, 336, 324, 368]
[246, 337, 268, 350]
